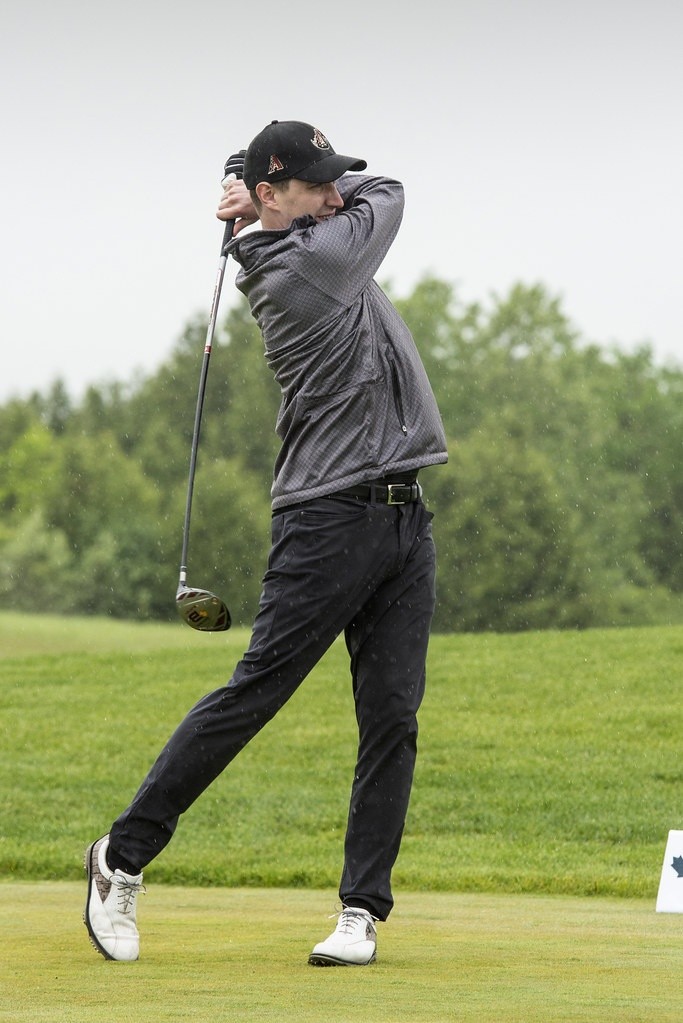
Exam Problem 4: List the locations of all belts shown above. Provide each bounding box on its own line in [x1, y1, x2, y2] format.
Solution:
[337, 481, 422, 505]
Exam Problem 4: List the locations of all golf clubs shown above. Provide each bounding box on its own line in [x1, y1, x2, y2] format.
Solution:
[173, 149, 248, 633]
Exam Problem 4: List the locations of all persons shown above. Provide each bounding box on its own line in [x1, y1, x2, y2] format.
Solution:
[83, 120, 450, 966]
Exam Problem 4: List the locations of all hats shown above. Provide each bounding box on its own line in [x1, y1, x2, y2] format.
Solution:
[243, 120, 367, 190]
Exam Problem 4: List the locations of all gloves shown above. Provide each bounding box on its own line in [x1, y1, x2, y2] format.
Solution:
[220, 153, 245, 191]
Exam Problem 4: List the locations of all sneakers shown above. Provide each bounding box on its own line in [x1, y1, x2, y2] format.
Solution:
[83, 832, 147, 962]
[308, 901, 379, 966]
